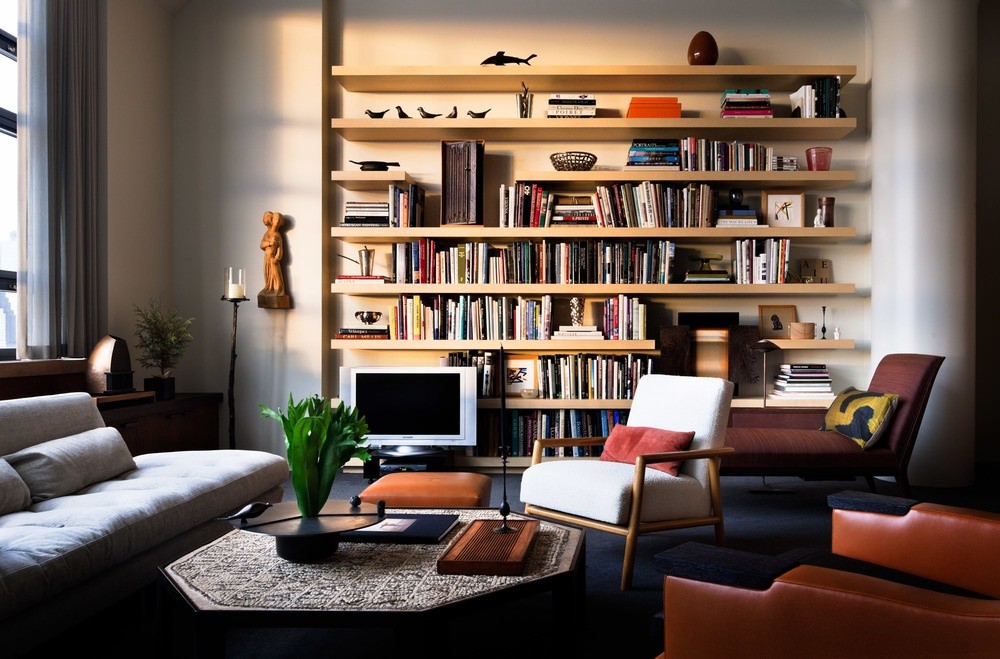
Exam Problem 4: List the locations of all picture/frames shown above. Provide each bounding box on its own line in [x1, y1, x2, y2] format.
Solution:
[761, 190, 806, 227]
[758, 305, 796, 339]
[495, 355, 539, 398]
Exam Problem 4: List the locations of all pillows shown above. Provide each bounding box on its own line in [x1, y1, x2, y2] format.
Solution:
[818, 386, 900, 450]
[600, 423, 696, 477]
[0, 457, 32, 517]
[0, 427, 137, 504]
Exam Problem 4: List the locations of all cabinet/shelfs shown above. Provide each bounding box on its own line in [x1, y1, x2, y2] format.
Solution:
[363, 449, 457, 485]
[331, 64, 858, 475]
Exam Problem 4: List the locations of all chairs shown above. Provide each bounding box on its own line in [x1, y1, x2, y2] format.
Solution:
[519, 374, 734, 592]
[652, 490, 1000, 659]
[715, 353, 946, 499]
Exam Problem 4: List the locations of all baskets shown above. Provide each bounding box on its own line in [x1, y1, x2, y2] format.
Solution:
[551, 151, 597, 172]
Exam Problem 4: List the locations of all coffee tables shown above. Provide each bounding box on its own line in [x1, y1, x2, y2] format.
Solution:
[157, 505, 586, 659]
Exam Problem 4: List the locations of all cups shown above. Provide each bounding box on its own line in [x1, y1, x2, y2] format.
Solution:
[818, 197, 835, 227]
[224, 268, 246, 298]
[806, 147, 833, 171]
[359, 249, 375, 276]
[729, 187, 743, 205]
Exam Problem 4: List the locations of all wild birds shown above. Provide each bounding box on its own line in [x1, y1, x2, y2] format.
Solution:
[364, 105, 492, 119]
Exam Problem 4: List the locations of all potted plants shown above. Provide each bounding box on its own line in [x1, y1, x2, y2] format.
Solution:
[132, 295, 195, 401]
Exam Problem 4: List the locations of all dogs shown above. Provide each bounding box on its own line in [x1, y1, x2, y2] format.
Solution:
[771, 314, 783, 331]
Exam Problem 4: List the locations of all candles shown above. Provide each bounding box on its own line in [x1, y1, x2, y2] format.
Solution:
[226, 284, 245, 298]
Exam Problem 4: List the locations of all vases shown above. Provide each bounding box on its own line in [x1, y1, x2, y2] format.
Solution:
[687, 31, 718, 65]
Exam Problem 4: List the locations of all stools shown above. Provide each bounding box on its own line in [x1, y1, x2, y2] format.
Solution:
[356, 470, 493, 508]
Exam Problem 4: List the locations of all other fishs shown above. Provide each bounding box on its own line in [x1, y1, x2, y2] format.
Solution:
[479, 51, 537, 67]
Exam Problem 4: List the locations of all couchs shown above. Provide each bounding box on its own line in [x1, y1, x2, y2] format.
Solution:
[0, 391, 291, 659]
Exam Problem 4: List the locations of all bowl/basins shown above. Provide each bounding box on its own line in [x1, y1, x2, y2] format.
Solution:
[355, 310, 382, 327]
[520, 389, 538, 399]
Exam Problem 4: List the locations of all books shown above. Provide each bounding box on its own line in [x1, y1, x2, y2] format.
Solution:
[335, 74, 842, 458]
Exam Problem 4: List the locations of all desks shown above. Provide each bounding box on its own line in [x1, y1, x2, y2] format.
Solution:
[100, 392, 224, 457]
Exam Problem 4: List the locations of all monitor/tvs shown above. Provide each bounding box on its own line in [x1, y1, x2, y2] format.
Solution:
[339, 366, 477, 456]
